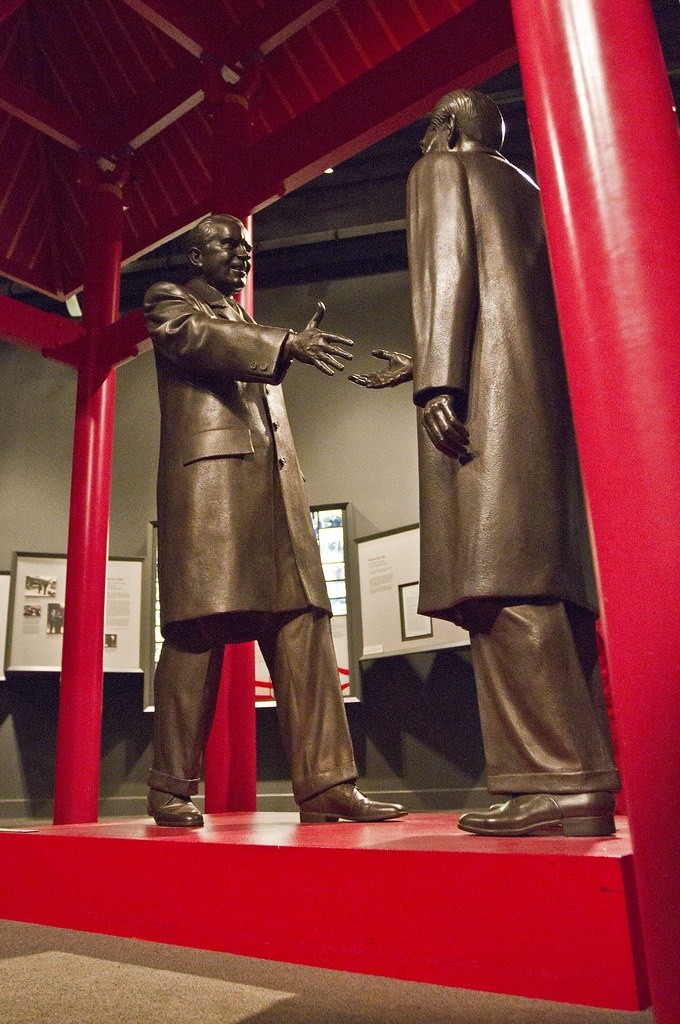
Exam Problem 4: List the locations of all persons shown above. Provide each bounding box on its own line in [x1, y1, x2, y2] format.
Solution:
[47, 609, 62, 634]
[38, 581, 47, 595]
[347, 87, 621, 839]
[141, 213, 410, 829]
